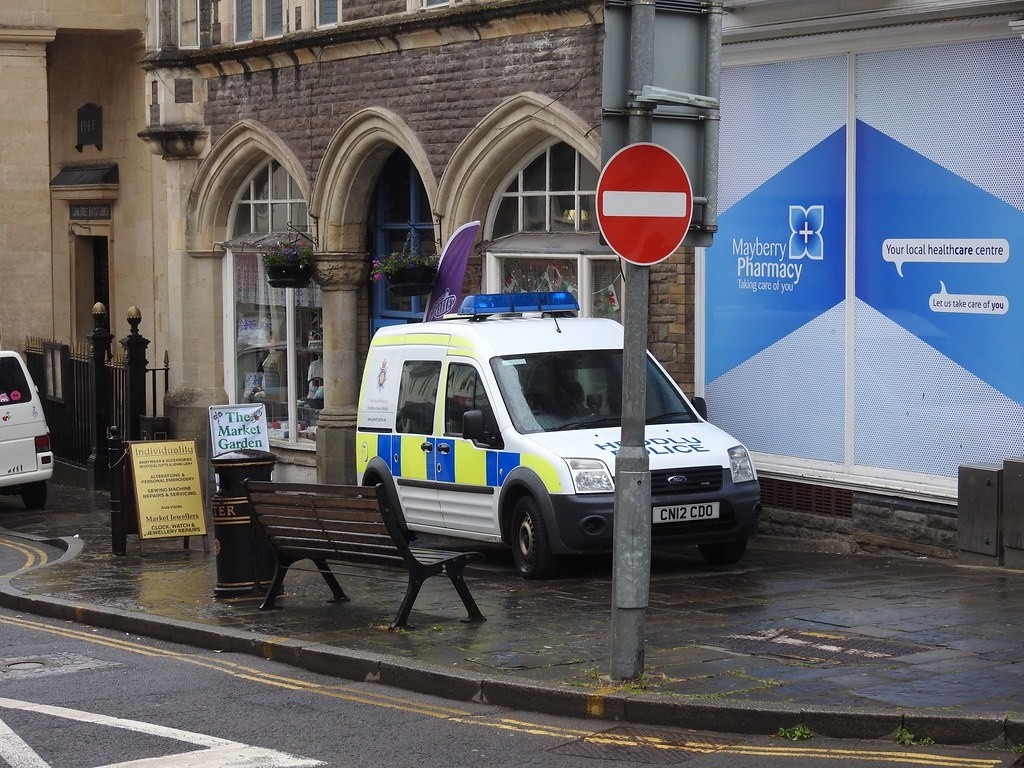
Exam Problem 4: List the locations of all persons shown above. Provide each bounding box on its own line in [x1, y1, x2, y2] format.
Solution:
[307, 354, 324, 398]
[552, 360, 584, 407]
[262, 349, 286, 401]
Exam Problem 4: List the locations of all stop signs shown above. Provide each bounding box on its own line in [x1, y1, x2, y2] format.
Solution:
[595, 141, 693, 267]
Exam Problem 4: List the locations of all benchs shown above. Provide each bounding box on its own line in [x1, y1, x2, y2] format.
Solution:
[243, 477, 488, 630]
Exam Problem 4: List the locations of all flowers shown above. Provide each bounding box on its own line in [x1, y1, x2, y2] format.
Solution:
[369, 252, 441, 283]
[259, 237, 315, 275]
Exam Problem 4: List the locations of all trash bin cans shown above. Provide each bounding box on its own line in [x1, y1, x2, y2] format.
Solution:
[206, 451, 287, 597]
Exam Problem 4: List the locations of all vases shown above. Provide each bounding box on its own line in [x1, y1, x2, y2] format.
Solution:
[385, 264, 438, 296]
[265, 262, 312, 288]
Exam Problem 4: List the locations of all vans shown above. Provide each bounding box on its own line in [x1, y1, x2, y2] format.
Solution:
[236, 339, 323, 406]
[0, 350, 56, 509]
[355, 290, 762, 582]
